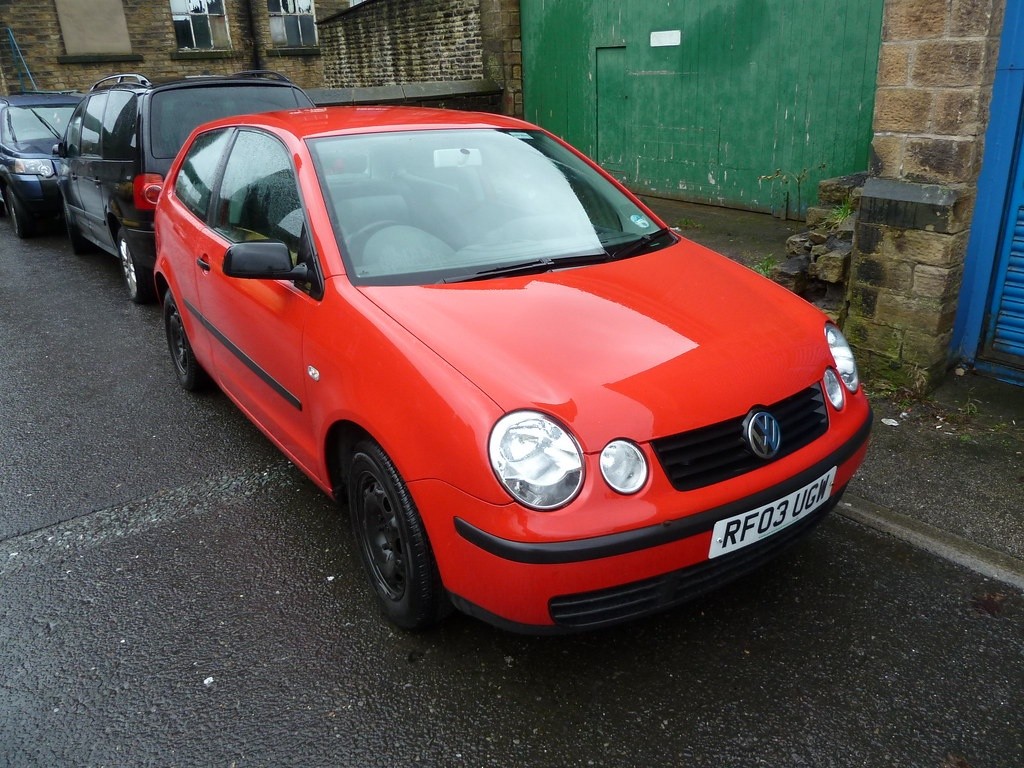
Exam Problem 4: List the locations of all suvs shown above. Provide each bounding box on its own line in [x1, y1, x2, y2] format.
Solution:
[47, 68, 325, 305]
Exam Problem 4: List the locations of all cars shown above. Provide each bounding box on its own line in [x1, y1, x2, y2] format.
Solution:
[149, 103, 877, 641]
[0, 87, 88, 239]
